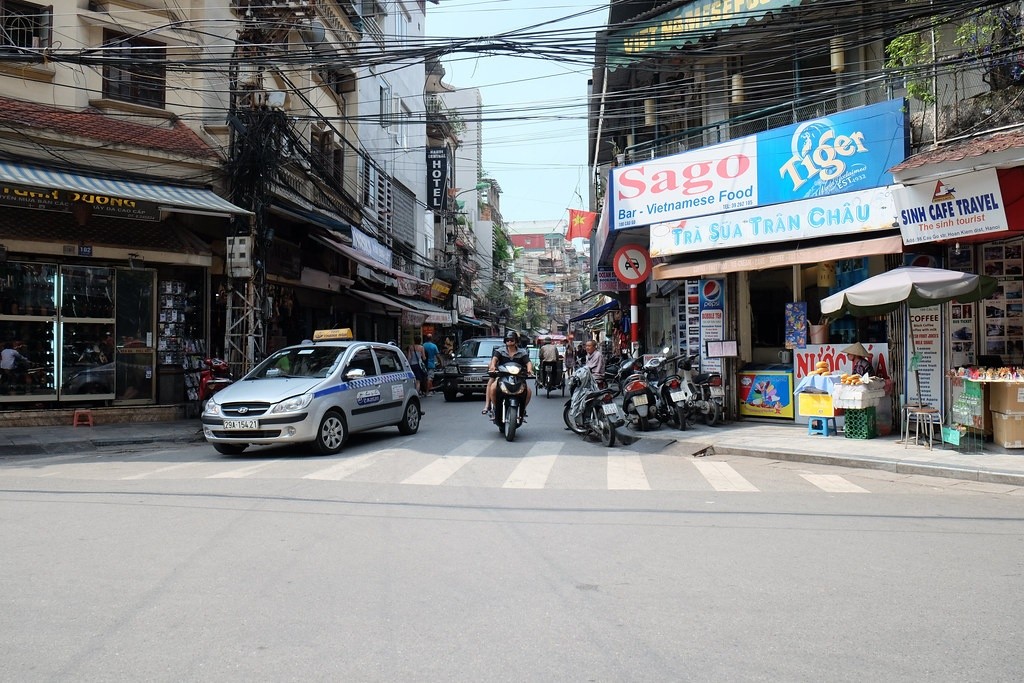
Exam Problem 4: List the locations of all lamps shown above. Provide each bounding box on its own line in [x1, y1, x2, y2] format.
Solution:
[643, 95, 654, 127]
[829, 10, 842, 73]
[731, 55, 742, 105]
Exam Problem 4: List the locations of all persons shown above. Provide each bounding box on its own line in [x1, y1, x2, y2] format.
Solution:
[408, 335, 426, 393]
[566, 345, 573, 379]
[540, 337, 560, 362]
[482, 331, 535, 424]
[841, 342, 876, 376]
[585, 340, 605, 377]
[423, 334, 440, 396]
[575, 345, 587, 363]
[0, 343, 31, 375]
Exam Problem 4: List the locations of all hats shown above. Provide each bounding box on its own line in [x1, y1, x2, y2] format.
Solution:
[841, 342, 870, 357]
[545, 337, 551, 344]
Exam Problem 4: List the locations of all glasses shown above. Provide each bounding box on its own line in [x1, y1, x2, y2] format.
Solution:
[506, 339, 514, 342]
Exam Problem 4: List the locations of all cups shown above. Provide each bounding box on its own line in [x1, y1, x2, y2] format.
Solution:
[778, 351, 790, 364]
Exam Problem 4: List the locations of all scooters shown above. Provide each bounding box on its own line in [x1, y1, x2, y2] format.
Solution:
[487, 363, 531, 443]
[562, 350, 726, 447]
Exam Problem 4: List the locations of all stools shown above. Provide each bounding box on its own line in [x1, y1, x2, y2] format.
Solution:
[900, 404, 928, 441]
[808, 416, 838, 437]
[905, 408, 945, 450]
[73, 410, 94, 428]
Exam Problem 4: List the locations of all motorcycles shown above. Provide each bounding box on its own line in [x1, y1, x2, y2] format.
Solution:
[201, 356, 236, 397]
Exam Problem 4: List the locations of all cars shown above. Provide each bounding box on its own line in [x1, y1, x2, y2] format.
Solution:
[201, 327, 427, 458]
[66, 360, 119, 394]
[527, 345, 542, 376]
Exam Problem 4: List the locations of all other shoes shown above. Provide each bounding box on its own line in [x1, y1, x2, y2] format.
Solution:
[426, 393, 432, 397]
[523, 418, 528, 423]
[488, 408, 496, 420]
[524, 410, 528, 417]
[482, 406, 491, 415]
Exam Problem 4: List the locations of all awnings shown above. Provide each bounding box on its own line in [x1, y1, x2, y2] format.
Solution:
[568, 300, 617, 334]
[0, 162, 254, 218]
[322, 236, 432, 285]
[348, 287, 421, 313]
[605, 0, 804, 71]
[386, 294, 453, 323]
[458, 316, 493, 329]
[651, 234, 903, 280]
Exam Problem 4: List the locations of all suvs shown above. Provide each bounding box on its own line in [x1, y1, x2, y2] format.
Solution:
[444, 339, 508, 402]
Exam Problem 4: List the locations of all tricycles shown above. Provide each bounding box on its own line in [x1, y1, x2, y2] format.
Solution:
[535, 360, 566, 401]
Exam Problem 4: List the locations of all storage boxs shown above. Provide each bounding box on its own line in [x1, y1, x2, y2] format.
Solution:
[797, 392, 836, 418]
[834, 384, 881, 409]
[991, 411, 1024, 449]
[988, 382, 1024, 415]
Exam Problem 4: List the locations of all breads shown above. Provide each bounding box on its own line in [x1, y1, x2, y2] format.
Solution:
[807, 361, 832, 376]
[840, 373, 863, 385]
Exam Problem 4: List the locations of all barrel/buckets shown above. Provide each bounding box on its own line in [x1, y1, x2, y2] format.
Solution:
[809, 325, 829, 344]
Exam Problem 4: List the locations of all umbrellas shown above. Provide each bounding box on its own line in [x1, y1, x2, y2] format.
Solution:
[819, 263, 998, 409]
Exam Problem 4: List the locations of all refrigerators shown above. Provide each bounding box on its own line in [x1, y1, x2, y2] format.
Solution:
[737, 362, 794, 419]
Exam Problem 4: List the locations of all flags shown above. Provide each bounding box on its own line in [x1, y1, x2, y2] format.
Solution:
[566, 210, 597, 240]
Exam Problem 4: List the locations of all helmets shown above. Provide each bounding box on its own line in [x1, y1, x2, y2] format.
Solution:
[503, 330, 519, 343]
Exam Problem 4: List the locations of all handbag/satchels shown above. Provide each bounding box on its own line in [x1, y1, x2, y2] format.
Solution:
[420, 368, 427, 384]
